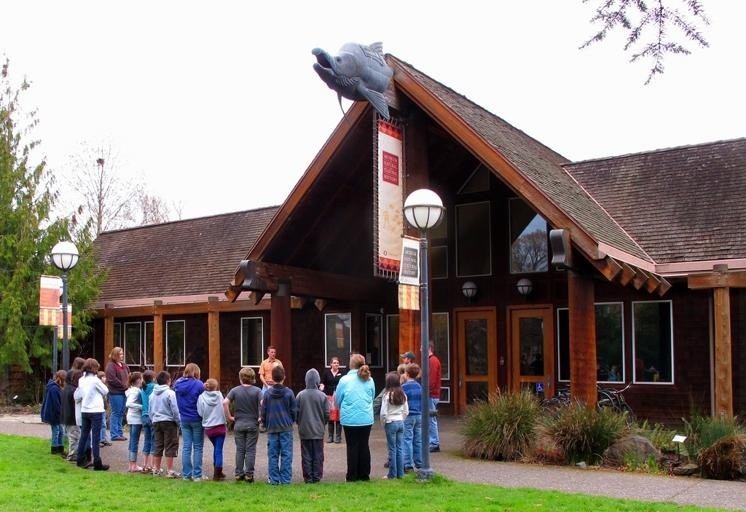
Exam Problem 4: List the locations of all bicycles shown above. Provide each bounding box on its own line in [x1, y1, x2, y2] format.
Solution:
[552, 381, 635, 417]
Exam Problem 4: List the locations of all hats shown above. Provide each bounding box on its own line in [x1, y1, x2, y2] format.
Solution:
[400, 351, 417, 361]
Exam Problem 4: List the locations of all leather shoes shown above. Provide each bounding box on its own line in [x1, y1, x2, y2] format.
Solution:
[111, 437, 124, 441]
[120, 434, 127, 440]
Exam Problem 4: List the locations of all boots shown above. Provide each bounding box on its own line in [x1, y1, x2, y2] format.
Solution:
[214, 463, 226, 478]
[213, 467, 224, 481]
[84, 462, 93, 468]
[335, 424, 342, 443]
[58, 446, 65, 452]
[94, 460, 110, 471]
[52, 446, 59, 454]
[326, 424, 334, 443]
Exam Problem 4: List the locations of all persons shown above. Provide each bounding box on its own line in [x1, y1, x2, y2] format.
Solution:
[380, 371, 409, 479]
[125, 372, 143, 473]
[106, 347, 130, 441]
[261, 366, 296, 485]
[41, 369, 66, 454]
[97, 371, 112, 447]
[319, 356, 344, 443]
[400, 363, 422, 472]
[148, 371, 181, 479]
[295, 368, 330, 483]
[398, 352, 422, 383]
[223, 368, 263, 482]
[420, 341, 441, 452]
[197, 379, 227, 481]
[173, 363, 205, 481]
[137, 370, 157, 474]
[335, 354, 375, 481]
[258, 346, 286, 395]
[65, 357, 86, 462]
[74, 358, 110, 470]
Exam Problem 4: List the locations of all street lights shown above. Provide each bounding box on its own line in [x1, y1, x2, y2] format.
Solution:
[51, 276, 63, 374]
[48, 242, 81, 373]
[402, 189, 447, 483]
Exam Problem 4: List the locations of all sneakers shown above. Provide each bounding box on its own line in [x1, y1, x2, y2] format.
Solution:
[246, 476, 253, 481]
[236, 475, 245, 479]
[167, 470, 181, 478]
[144, 466, 152, 472]
[99, 443, 104, 447]
[131, 467, 142, 472]
[431, 446, 439, 452]
[103, 439, 111, 445]
[194, 475, 208, 481]
[153, 470, 164, 475]
[152, 467, 163, 472]
